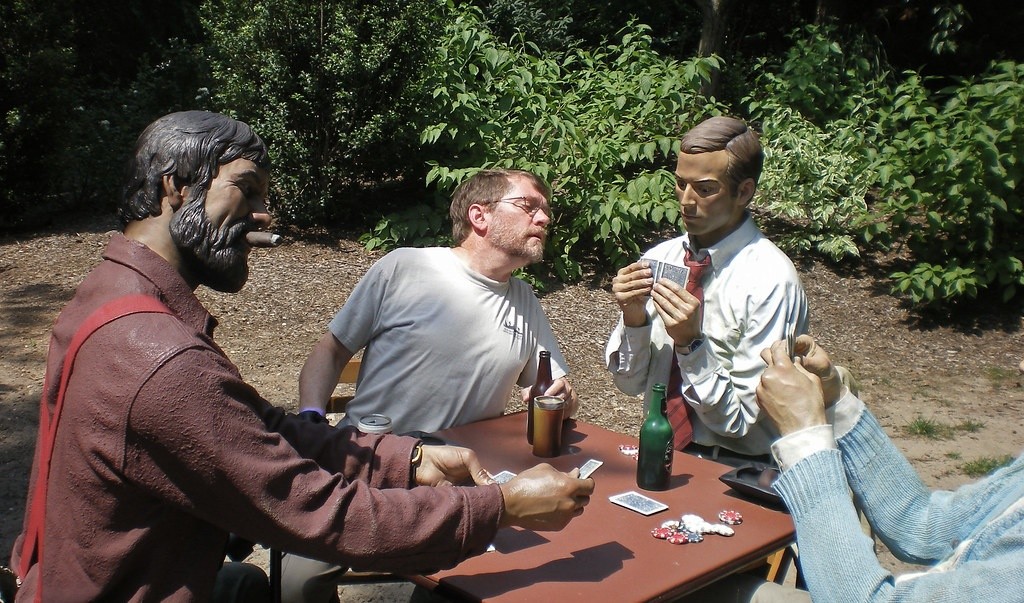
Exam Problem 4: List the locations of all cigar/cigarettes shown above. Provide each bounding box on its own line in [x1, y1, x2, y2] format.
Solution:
[246, 232, 282, 249]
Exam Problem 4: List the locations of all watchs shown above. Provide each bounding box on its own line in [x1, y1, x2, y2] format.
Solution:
[675, 334, 706, 355]
[410, 440, 423, 486]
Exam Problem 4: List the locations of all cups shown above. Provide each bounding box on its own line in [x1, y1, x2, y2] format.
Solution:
[533, 396, 564, 458]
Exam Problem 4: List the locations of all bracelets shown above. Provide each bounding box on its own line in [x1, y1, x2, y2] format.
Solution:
[299, 408, 326, 417]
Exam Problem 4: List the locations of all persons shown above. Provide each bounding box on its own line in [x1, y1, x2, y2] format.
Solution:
[605, 116, 808, 478]
[280, 169, 579, 602]
[0, 110, 596, 603]
[755, 333, 1023, 602]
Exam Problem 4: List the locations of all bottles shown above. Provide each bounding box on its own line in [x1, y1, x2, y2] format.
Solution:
[527, 351, 552, 444]
[636, 383, 674, 492]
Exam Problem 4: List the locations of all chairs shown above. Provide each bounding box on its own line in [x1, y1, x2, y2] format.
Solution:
[269, 362, 420, 603]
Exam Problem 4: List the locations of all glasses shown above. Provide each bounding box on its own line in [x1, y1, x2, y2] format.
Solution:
[479, 196, 555, 227]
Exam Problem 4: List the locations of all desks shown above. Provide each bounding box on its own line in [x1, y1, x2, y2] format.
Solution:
[428, 410, 799, 603]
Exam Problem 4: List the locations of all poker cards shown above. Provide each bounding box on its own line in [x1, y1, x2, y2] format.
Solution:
[785, 322, 797, 357]
[609, 490, 669, 516]
[578, 459, 604, 480]
[473, 470, 518, 488]
[636, 257, 691, 299]
